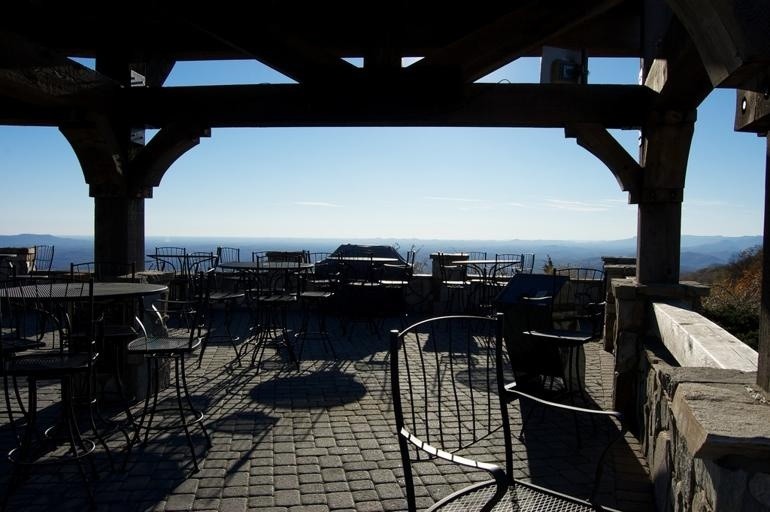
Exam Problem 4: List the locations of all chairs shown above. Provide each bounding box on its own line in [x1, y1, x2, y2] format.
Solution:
[0, 244, 629, 512]
